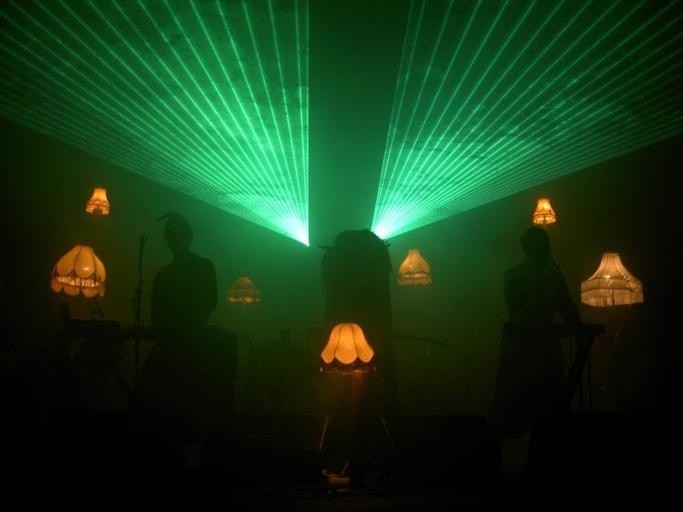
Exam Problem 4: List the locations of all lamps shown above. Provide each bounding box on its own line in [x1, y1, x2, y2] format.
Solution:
[317, 319, 379, 381]
[531, 197, 557, 226]
[47, 186, 111, 301]
[578, 250, 645, 310]
[395, 248, 432, 287]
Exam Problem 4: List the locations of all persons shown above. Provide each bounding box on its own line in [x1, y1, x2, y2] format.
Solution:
[151, 213, 218, 341]
[490, 226, 585, 426]
